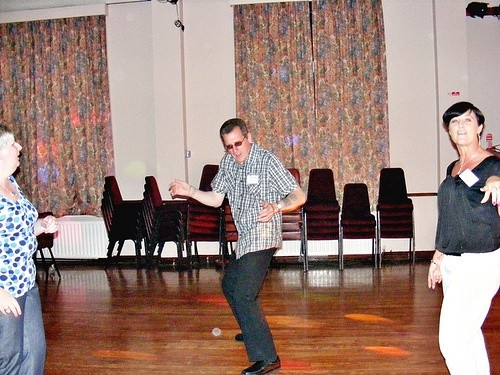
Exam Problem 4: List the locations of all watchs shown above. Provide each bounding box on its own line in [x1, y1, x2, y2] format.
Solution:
[277, 201, 282, 212]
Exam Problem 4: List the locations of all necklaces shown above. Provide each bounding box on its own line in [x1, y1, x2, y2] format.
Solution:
[458, 147, 481, 175]
[0, 184, 16, 196]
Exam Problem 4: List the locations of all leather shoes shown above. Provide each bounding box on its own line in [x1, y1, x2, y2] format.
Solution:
[242, 355, 281, 375]
[235, 334, 245, 341]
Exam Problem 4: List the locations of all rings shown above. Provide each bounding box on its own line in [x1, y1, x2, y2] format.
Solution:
[491, 185, 497, 190]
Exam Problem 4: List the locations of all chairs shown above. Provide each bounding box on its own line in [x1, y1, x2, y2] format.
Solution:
[100, 164, 238, 273]
[281, 168, 416, 272]
[33, 212, 61, 283]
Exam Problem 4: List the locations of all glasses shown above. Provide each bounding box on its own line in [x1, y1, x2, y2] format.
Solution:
[225, 133, 247, 149]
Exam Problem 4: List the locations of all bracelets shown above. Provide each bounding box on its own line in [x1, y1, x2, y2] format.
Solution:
[431, 260, 439, 265]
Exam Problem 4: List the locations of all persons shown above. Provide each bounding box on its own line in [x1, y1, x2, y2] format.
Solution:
[428, 101, 500, 375]
[0, 124, 58, 374]
[168, 118, 306, 375]
